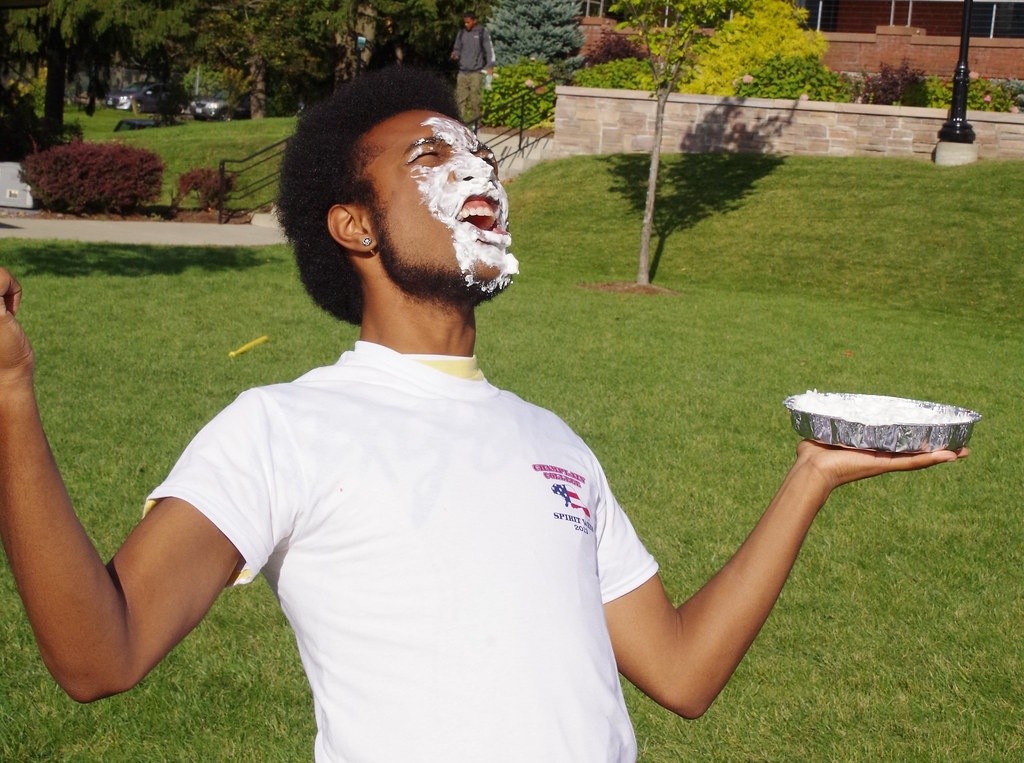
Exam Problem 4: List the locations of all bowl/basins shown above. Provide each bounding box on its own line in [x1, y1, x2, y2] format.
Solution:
[782, 391, 983, 456]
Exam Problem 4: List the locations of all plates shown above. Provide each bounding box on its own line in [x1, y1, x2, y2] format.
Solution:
[485, 73, 492, 90]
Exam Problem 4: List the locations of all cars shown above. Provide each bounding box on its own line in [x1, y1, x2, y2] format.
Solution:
[190, 96, 251, 121]
[132, 83, 186, 115]
[105, 80, 156, 110]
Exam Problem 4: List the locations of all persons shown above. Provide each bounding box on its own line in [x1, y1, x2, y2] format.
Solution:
[450, 11, 496, 121]
[0, 67, 971, 763]
